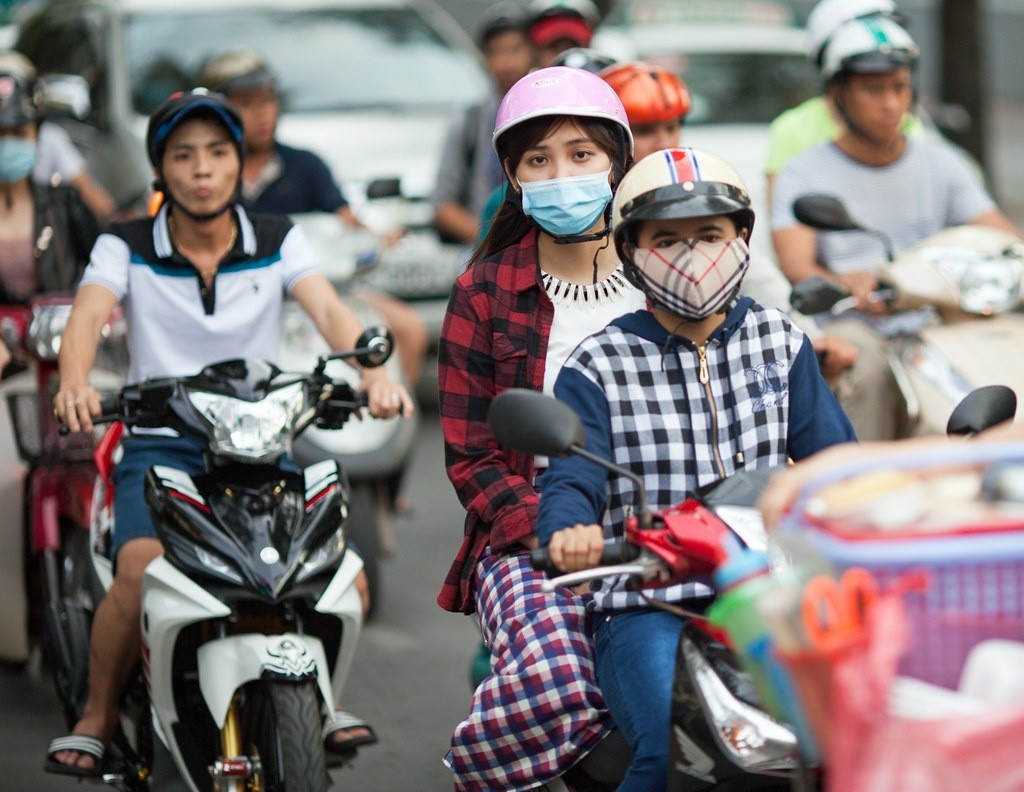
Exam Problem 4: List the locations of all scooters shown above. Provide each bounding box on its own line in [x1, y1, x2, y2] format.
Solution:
[489, 383, 1023, 792]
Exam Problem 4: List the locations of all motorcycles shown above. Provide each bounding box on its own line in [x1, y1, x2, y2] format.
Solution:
[785, 190, 1023, 436]
[0, 175, 415, 792]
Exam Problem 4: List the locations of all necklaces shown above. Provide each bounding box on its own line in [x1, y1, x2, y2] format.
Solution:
[168, 216, 238, 278]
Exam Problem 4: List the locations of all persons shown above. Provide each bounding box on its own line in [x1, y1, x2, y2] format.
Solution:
[42, 85, 414, 780]
[430, 0, 695, 307]
[436, 66, 670, 792]
[190, 47, 424, 514]
[0, 48, 129, 363]
[536, 147, 859, 792]
[766, 0, 1024, 443]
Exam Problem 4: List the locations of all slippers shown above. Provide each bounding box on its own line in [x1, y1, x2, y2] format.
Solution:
[320, 708, 380, 750]
[44, 734, 106, 777]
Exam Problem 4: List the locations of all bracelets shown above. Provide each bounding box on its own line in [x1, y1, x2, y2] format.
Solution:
[356, 363, 387, 376]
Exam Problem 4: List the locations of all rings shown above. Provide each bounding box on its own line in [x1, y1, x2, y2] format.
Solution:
[75, 396, 86, 402]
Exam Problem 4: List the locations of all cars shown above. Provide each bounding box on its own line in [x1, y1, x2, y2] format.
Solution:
[0, 1, 504, 400]
[104, 1, 510, 412]
[587, 20, 988, 310]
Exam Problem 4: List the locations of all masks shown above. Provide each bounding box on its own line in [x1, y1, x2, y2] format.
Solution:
[515, 159, 616, 240]
[631, 235, 751, 319]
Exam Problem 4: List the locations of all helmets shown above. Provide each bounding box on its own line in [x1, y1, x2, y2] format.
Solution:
[199, 49, 265, 91]
[145, 87, 248, 165]
[554, 47, 618, 74]
[490, 64, 639, 160]
[818, 16, 920, 89]
[803, 0, 911, 67]
[0, 46, 48, 128]
[609, 146, 755, 271]
[527, 6, 595, 45]
[597, 60, 693, 122]
